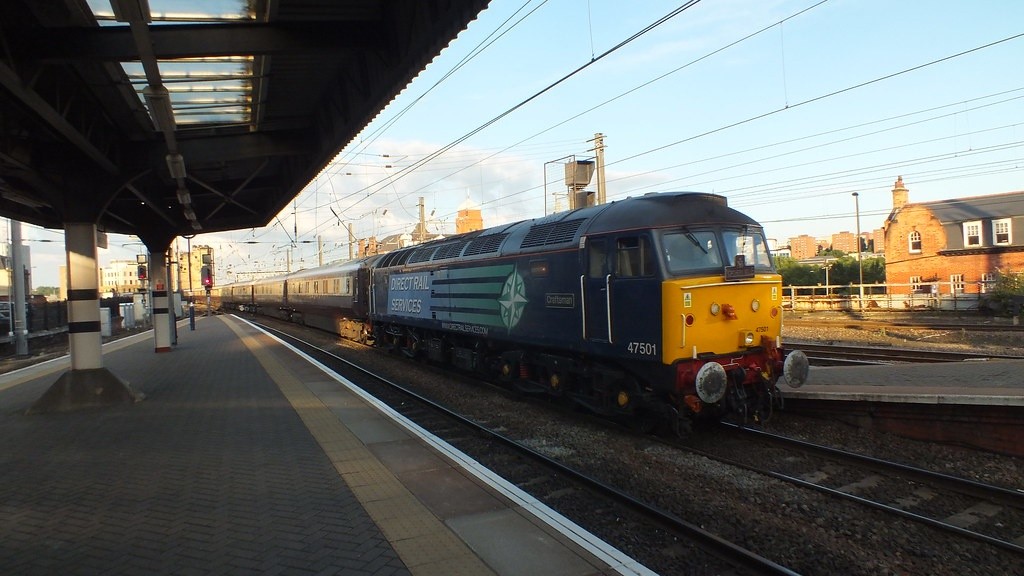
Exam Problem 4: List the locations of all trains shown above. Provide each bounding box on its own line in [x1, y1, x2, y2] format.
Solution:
[219, 190, 810, 445]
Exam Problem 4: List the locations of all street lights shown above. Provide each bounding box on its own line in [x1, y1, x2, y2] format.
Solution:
[851, 192, 865, 311]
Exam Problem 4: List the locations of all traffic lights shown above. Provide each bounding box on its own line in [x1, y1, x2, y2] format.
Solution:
[204, 275, 214, 290]
[199, 266, 210, 285]
[137, 265, 146, 279]
